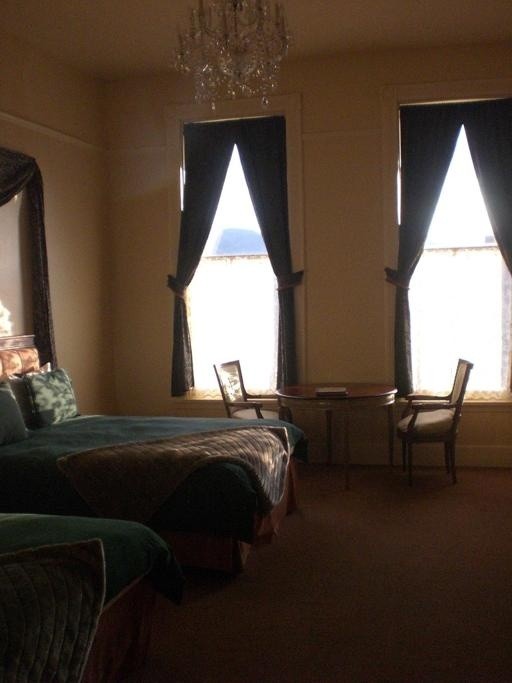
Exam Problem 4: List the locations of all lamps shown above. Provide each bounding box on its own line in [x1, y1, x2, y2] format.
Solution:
[168, 0, 294, 118]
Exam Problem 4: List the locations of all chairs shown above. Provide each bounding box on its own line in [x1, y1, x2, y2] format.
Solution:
[212, 357, 279, 419]
[396, 358, 474, 485]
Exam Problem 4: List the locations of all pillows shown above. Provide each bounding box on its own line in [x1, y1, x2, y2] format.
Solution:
[0, 382, 35, 446]
[23, 366, 78, 427]
[8, 362, 52, 410]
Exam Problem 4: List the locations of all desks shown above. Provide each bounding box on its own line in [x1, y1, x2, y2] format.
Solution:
[272, 382, 399, 473]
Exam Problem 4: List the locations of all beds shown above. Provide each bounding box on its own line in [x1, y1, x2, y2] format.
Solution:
[1, 511, 179, 682]
[0, 330, 306, 576]
